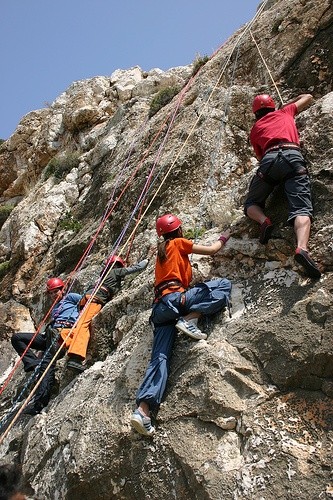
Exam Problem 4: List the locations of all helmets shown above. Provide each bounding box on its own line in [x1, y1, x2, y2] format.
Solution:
[46, 278, 64, 291]
[156, 214, 182, 237]
[107, 255, 125, 267]
[252, 94, 275, 113]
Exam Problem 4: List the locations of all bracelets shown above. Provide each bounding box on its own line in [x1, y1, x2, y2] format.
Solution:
[218, 235, 226, 242]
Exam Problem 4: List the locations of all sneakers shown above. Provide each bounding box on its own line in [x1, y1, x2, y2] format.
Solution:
[294, 247, 321, 280]
[46, 328, 60, 354]
[130, 410, 155, 436]
[175, 317, 207, 340]
[65, 355, 89, 375]
[29, 401, 44, 416]
[259, 216, 274, 245]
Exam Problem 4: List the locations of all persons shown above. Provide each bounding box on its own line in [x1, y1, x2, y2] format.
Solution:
[9, 278, 84, 406]
[59, 245, 158, 372]
[241, 94, 320, 280]
[130, 214, 233, 436]
